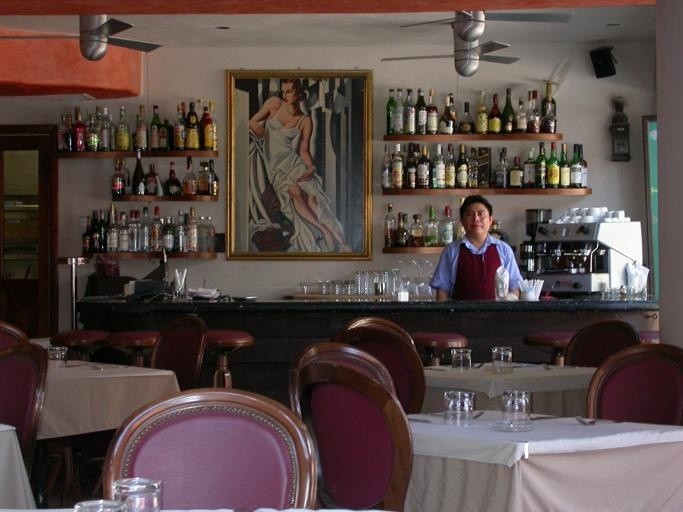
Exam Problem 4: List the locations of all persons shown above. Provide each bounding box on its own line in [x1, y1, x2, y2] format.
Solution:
[430, 195, 524, 302]
[249, 78, 353, 252]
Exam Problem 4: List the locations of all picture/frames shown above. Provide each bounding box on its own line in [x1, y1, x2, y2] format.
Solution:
[225, 69, 374, 260]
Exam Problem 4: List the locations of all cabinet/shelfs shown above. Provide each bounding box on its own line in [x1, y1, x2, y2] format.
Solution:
[383, 131, 594, 257]
[62, 147, 221, 263]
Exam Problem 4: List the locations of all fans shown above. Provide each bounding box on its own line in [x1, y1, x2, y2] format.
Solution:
[400, 10, 573, 42]
[0, 15, 163, 62]
[380, 28, 522, 77]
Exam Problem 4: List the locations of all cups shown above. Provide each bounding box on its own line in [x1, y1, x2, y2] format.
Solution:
[45, 345, 68, 369]
[73, 498, 127, 512]
[110, 476, 163, 512]
[172, 272, 187, 303]
[295, 256, 436, 297]
[491, 346, 513, 374]
[441, 389, 477, 429]
[451, 348, 472, 376]
[501, 389, 533, 430]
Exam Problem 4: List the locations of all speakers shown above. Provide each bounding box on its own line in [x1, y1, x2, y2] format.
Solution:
[589, 47, 617, 78]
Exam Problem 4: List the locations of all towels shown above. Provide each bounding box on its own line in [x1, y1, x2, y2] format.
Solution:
[494, 264, 509, 301]
[174, 268, 188, 297]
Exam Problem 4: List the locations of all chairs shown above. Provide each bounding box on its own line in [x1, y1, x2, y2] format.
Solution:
[288, 341, 396, 420]
[586, 343, 683, 427]
[1, 343, 49, 481]
[348, 317, 416, 349]
[333, 324, 426, 415]
[148, 313, 208, 391]
[300, 361, 412, 512]
[0, 321, 29, 351]
[563, 320, 640, 370]
[102, 388, 318, 512]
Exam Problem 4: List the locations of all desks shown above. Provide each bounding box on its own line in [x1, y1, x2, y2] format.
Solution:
[75, 299, 662, 405]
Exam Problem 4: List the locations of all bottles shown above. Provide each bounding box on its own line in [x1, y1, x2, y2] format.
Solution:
[91, 209, 100, 252]
[393, 89, 404, 134]
[510, 156, 523, 188]
[106, 204, 118, 252]
[547, 142, 561, 188]
[164, 160, 183, 195]
[196, 99, 203, 124]
[380, 142, 391, 189]
[133, 148, 146, 195]
[121, 159, 130, 196]
[610, 95, 631, 160]
[468, 147, 479, 189]
[524, 148, 537, 187]
[536, 142, 548, 189]
[542, 80, 557, 134]
[431, 148, 444, 188]
[438, 206, 457, 246]
[160, 117, 174, 150]
[571, 144, 582, 189]
[129, 209, 138, 252]
[207, 216, 216, 251]
[528, 90, 532, 114]
[99, 105, 110, 151]
[515, 97, 527, 132]
[426, 88, 438, 134]
[414, 88, 426, 134]
[118, 212, 130, 252]
[172, 218, 188, 252]
[435, 145, 446, 187]
[183, 154, 196, 194]
[153, 174, 164, 196]
[198, 216, 208, 251]
[559, 143, 571, 189]
[132, 114, 139, 151]
[145, 164, 157, 196]
[85, 113, 98, 151]
[178, 208, 185, 224]
[57, 113, 68, 151]
[489, 93, 502, 133]
[395, 212, 410, 247]
[456, 144, 468, 188]
[502, 87, 515, 132]
[111, 158, 124, 196]
[391, 143, 404, 189]
[206, 162, 219, 195]
[424, 204, 438, 246]
[502, 147, 509, 170]
[201, 106, 214, 150]
[386, 88, 397, 135]
[151, 206, 163, 252]
[188, 216, 198, 252]
[449, 93, 456, 121]
[403, 89, 415, 136]
[445, 144, 457, 188]
[497, 221, 508, 242]
[405, 144, 416, 189]
[190, 207, 195, 218]
[579, 144, 588, 188]
[116, 104, 129, 152]
[544, 84, 554, 134]
[109, 110, 116, 151]
[135, 210, 143, 252]
[475, 90, 488, 134]
[402, 212, 408, 230]
[181, 102, 187, 123]
[97, 208, 107, 252]
[383, 203, 396, 247]
[439, 96, 455, 134]
[495, 152, 507, 188]
[150, 105, 162, 151]
[209, 159, 214, 194]
[209, 101, 218, 150]
[459, 101, 473, 134]
[174, 103, 186, 150]
[73, 112, 85, 151]
[75, 106, 81, 114]
[487, 220, 502, 242]
[529, 90, 541, 133]
[409, 214, 424, 247]
[186, 102, 200, 150]
[66, 113, 74, 150]
[456, 196, 470, 244]
[136, 104, 147, 150]
[198, 162, 208, 195]
[163, 216, 174, 252]
[141, 207, 150, 253]
[417, 146, 432, 189]
[81, 215, 91, 256]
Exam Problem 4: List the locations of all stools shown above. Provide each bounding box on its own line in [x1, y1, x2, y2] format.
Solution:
[330, 333, 342, 342]
[204, 328, 255, 389]
[412, 332, 470, 366]
[49, 329, 111, 507]
[524, 330, 578, 367]
[112, 329, 159, 367]
